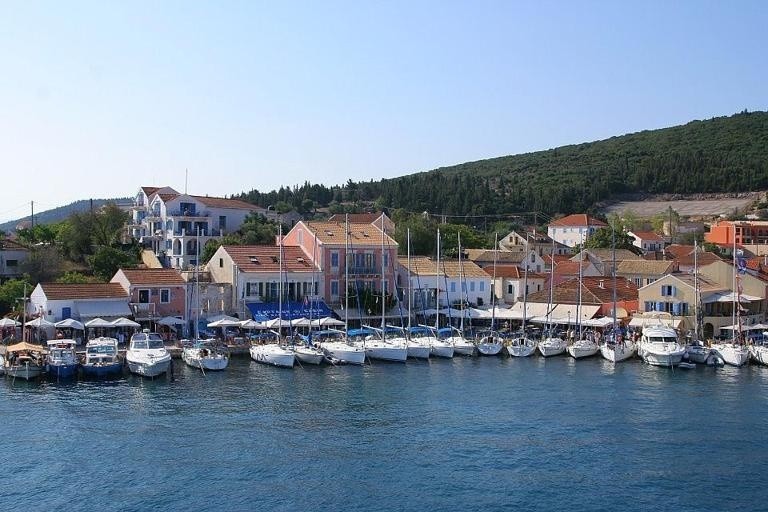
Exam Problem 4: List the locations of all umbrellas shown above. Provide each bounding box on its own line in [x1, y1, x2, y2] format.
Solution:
[83, 317, 115, 329]
[24, 317, 52, 342]
[238, 317, 267, 340]
[111, 317, 141, 332]
[262, 316, 291, 331]
[154, 315, 186, 326]
[206, 317, 241, 341]
[53, 318, 85, 329]
[206, 314, 239, 324]
[415, 306, 623, 331]
[0, 316, 22, 329]
[313, 315, 346, 337]
[290, 316, 316, 335]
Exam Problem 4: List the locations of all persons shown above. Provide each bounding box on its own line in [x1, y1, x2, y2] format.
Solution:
[568, 327, 640, 349]
[0, 329, 323, 348]
[199, 347, 212, 359]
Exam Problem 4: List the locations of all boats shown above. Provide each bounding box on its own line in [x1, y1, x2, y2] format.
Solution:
[180, 225, 229, 371]
[44, 339, 80, 378]
[81, 336, 124, 377]
[125, 328, 171, 378]
[0, 342, 46, 380]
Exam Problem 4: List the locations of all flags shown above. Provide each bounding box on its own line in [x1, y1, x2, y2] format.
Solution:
[303, 295, 308, 306]
